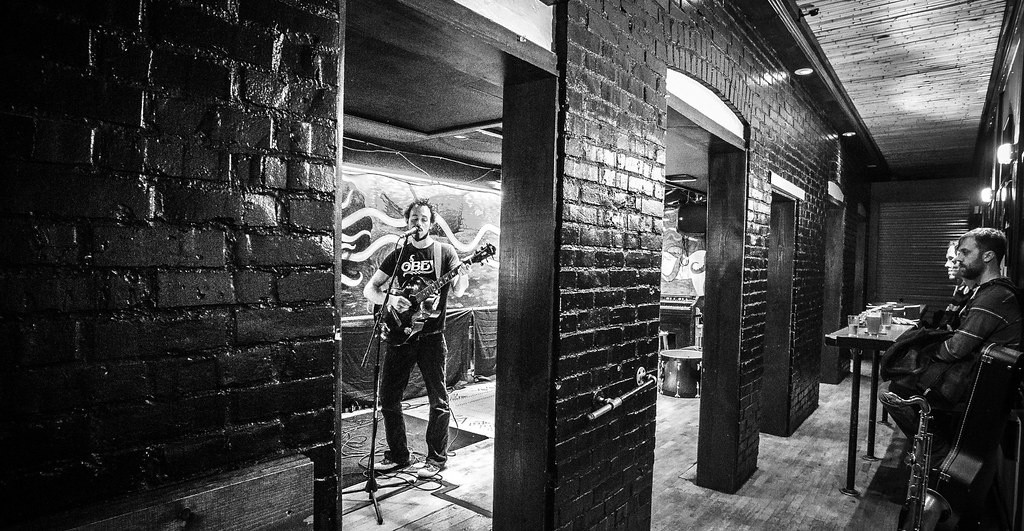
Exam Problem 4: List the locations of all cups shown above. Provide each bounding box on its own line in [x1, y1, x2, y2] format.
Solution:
[881, 306, 893, 330]
[866, 310, 882, 337]
[848, 316, 860, 337]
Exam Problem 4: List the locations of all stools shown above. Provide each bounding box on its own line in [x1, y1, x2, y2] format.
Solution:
[658, 333, 669, 394]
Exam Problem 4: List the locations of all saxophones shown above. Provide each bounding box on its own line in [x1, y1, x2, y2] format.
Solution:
[876, 379, 953, 531]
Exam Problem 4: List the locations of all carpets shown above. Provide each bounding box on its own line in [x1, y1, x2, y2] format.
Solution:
[339, 410, 488, 490]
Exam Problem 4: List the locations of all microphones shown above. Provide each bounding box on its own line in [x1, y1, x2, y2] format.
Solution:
[400, 226, 419, 238]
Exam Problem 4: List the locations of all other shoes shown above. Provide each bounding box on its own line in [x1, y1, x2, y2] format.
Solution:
[417, 461, 441, 477]
[374, 458, 408, 473]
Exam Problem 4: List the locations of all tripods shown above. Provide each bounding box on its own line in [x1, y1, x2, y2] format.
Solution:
[341, 236, 414, 525]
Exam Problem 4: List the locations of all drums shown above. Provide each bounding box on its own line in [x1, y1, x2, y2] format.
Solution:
[660, 349, 703, 398]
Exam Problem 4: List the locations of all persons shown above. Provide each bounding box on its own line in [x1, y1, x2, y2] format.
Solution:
[888, 227, 1024, 471]
[362, 202, 468, 479]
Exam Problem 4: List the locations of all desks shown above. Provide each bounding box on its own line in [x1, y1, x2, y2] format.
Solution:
[824, 300, 929, 497]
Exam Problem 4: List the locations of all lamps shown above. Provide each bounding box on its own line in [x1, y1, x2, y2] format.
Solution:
[996, 143, 1019, 165]
[981, 187, 998, 202]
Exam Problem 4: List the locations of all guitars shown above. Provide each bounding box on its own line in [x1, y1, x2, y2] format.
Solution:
[373, 241, 496, 347]
